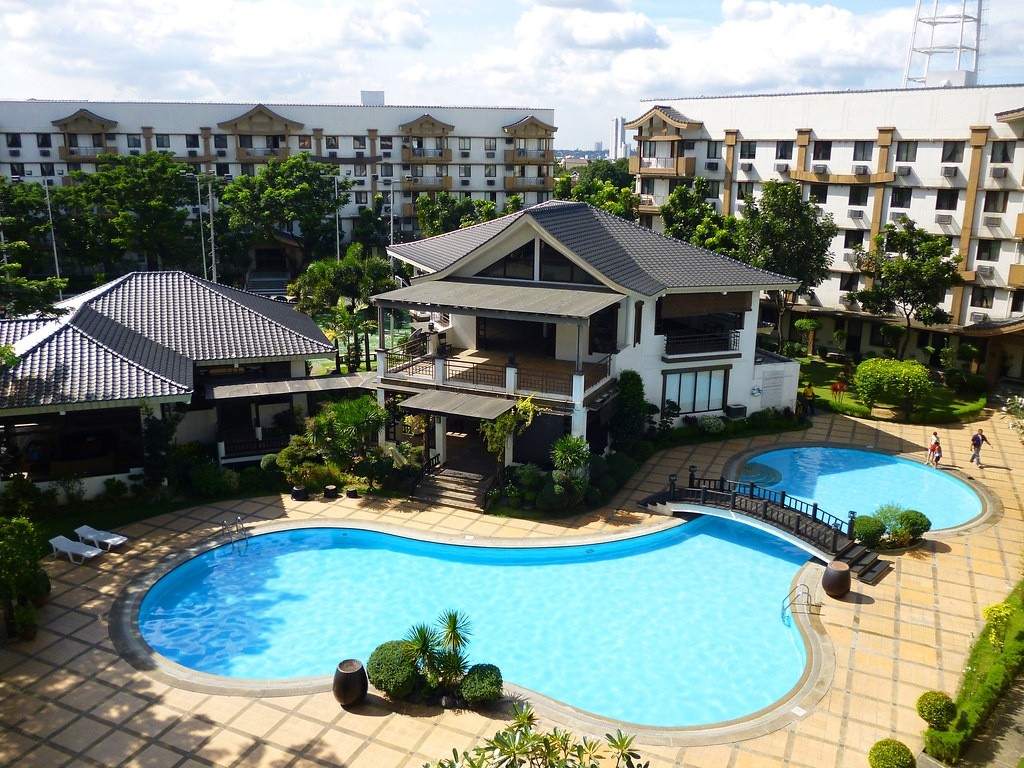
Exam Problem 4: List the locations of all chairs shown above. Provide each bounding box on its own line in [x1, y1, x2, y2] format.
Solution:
[49, 535, 103, 565]
[74, 524, 128, 553]
[438, 332, 452, 355]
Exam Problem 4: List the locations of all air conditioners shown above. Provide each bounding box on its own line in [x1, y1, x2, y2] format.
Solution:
[705, 162, 1007, 323]
[413, 177, 420, 184]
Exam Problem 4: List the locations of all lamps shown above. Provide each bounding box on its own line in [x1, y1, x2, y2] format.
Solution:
[755, 356, 766, 363]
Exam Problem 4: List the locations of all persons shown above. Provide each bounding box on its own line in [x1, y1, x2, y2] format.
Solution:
[803, 383, 815, 415]
[28, 442, 47, 462]
[924, 432, 940, 465]
[970, 429, 993, 468]
[934, 442, 942, 469]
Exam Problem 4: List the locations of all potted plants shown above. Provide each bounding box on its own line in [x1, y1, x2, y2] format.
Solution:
[486, 460, 542, 510]
[366, 609, 504, 711]
